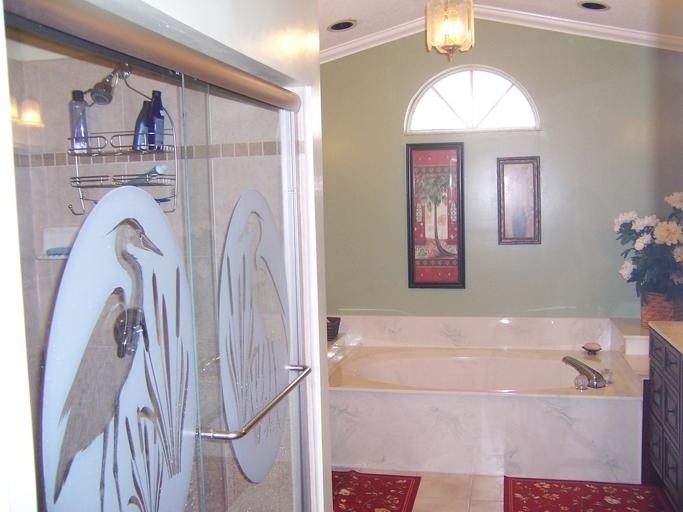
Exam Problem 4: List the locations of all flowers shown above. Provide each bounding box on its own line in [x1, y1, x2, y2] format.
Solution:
[614, 191, 683, 303]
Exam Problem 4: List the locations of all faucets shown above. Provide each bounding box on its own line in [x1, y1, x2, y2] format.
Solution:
[562, 356, 607, 389]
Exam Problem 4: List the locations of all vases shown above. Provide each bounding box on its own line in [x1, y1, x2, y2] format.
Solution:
[640, 289, 682, 329]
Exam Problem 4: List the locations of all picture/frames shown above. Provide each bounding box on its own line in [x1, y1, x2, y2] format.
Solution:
[496, 157, 541, 245]
[406, 141, 465, 288]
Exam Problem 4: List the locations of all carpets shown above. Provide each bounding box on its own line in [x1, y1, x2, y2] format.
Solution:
[332, 470, 422, 512]
[504, 476, 674, 512]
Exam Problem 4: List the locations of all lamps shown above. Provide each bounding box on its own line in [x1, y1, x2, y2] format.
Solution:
[426, 0, 474, 61]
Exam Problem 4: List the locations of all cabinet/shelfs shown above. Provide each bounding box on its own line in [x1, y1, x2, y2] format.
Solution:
[67, 61, 177, 215]
[647, 321, 683, 512]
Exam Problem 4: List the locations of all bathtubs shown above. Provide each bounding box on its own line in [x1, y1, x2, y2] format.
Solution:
[325, 344, 643, 486]
